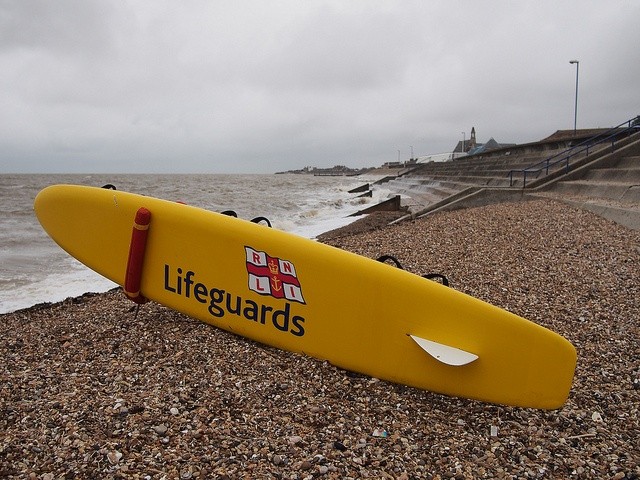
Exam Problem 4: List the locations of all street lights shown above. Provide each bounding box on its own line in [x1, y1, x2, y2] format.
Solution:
[569, 60, 579, 136]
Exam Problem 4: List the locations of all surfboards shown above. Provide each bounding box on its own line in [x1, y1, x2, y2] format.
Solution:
[34, 184, 577, 410]
